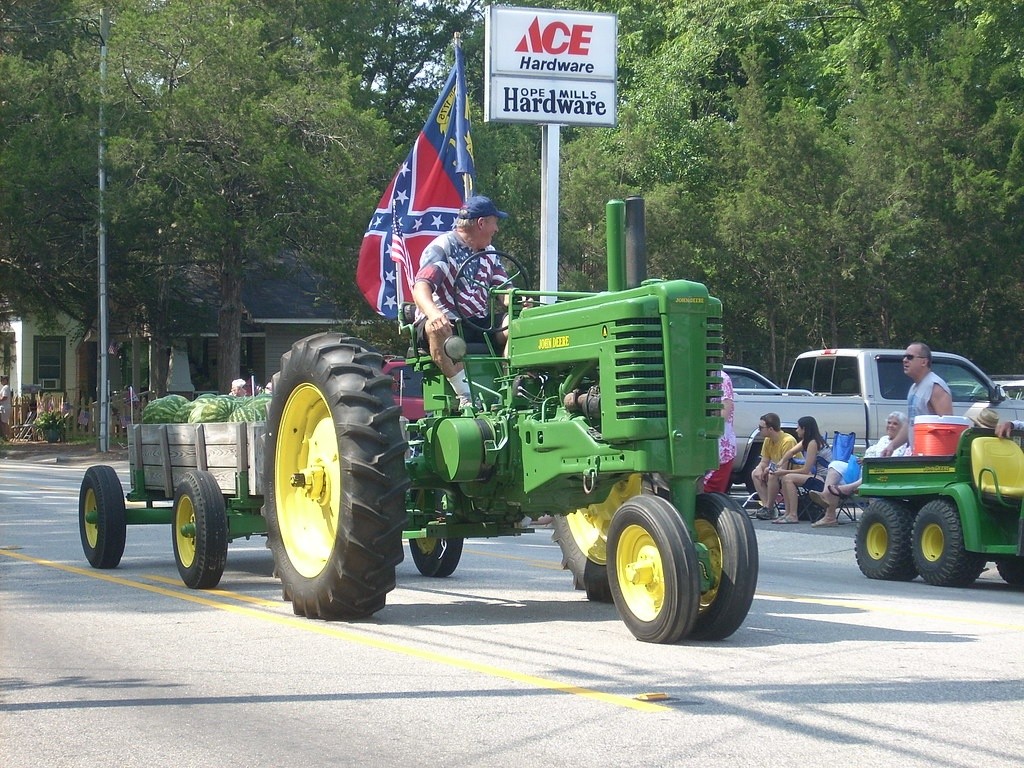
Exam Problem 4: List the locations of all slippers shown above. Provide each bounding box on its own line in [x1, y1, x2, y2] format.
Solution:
[771, 514, 800, 525]
[809, 489, 830, 509]
[811, 518, 840, 528]
[827, 484, 851, 497]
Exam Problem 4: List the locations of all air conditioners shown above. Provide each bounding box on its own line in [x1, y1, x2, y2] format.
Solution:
[42, 379, 61, 389]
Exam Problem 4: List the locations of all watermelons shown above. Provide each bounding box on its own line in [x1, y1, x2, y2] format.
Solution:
[141, 393, 273, 423]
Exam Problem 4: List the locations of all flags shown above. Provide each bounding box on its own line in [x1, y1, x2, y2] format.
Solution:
[354, 44, 475, 318]
[108, 341, 120, 356]
[120, 416, 131, 428]
[62, 404, 72, 415]
[77, 410, 90, 426]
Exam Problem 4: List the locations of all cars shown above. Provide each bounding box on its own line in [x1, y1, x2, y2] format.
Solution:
[721, 365, 787, 396]
[945, 375, 1024, 403]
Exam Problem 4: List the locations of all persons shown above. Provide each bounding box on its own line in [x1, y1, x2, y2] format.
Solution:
[414, 195, 533, 409]
[228, 378, 247, 397]
[748, 413, 811, 520]
[0, 375, 10, 442]
[265, 383, 272, 395]
[995, 420, 1024, 440]
[703, 369, 737, 494]
[809, 411, 908, 527]
[880, 342, 953, 457]
[769, 415, 832, 524]
[966, 408, 1000, 429]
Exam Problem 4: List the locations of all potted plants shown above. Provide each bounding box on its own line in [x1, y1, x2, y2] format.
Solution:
[33, 410, 71, 443]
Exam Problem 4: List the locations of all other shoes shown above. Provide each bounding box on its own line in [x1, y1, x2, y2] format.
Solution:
[754, 506, 778, 520]
[455, 391, 481, 411]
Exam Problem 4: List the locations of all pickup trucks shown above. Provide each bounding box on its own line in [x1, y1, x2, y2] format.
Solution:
[720, 348, 1024, 501]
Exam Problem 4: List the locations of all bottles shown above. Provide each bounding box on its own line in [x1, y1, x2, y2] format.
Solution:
[769, 460, 777, 472]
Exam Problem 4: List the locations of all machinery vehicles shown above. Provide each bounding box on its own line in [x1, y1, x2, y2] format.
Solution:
[77, 199, 759, 643]
[854, 427, 1024, 586]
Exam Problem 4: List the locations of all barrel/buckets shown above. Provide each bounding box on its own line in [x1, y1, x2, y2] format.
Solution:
[914, 415, 970, 456]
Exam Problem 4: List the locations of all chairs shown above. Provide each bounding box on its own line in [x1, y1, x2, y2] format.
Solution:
[802, 379, 812, 387]
[970, 437, 1024, 503]
[398, 303, 501, 364]
[841, 378, 858, 390]
[743, 426, 867, 524]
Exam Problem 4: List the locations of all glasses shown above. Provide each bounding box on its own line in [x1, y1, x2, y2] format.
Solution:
[758, 425, 771, 430]
[902, 353, 927, 360]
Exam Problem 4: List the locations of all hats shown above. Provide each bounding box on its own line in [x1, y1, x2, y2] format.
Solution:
[457, 195, 511, 219]
[967, 408, 1000, 430]
